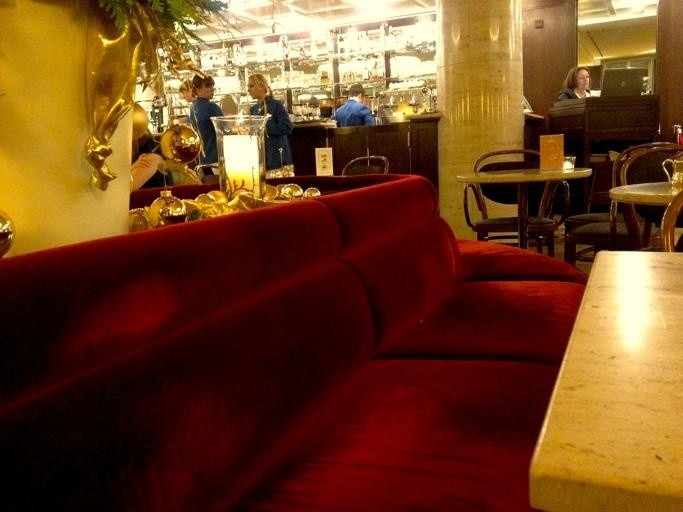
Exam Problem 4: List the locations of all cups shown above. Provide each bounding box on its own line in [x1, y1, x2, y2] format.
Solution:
[662, 159, 682, 187]
[211, 115, 268, 203]
[563, 156, 576, 173]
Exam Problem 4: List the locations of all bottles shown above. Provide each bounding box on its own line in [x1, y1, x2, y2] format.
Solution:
[676, 128, 683, 145]
[320, 94, 374, 106]
[342, 71, 354, 81]
[293, 102, 322, 120]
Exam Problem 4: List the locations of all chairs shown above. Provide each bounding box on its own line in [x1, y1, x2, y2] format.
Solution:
[338, 152, 391, 178]
[452, 137, 681, 266]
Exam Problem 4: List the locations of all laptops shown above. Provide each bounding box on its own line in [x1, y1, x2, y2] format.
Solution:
[600, 68, 645, 97]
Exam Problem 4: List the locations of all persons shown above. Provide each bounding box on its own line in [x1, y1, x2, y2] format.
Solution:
[246, 73, 296, 177]
[178, 79, 199, 136]
[556, 66, 592, 101]
[191, 73, 224, 176]
[329, 83, 374, 129]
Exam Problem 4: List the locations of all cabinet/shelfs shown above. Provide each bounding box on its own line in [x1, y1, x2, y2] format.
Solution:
[130, 40, 435, 123]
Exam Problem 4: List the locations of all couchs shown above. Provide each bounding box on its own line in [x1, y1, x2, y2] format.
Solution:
[0, 197, 562, 511]
[315, 173, 587, 370]
[128, 173, 589, 286]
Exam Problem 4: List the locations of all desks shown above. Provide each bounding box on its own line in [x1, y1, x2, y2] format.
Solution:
[521, 246, 682, 509]
